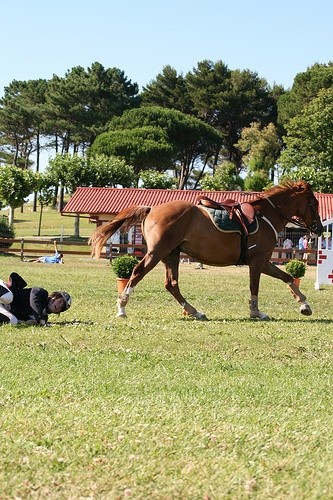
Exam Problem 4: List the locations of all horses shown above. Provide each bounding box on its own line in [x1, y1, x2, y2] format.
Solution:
[87, 174, 324, 321]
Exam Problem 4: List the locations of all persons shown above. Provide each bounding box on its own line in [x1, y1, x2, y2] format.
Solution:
[283, 237, 293, 259]
[0, 273, 72, 328]
[24, 241, 63, 264]
[180, 256, 190, 264]
[299, 233, 333, 262]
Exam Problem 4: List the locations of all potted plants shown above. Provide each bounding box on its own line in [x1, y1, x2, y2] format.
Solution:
[285, 259, 307, 287]
[111, 253, 137, 293]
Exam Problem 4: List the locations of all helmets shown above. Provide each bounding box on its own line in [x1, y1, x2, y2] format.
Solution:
[52, 291, 71, 312]
